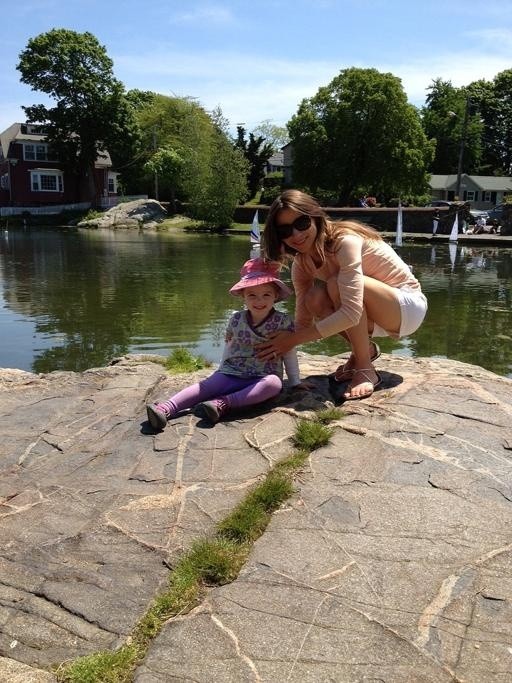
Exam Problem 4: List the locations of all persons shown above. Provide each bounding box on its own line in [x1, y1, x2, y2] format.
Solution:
[431, 207, 440, 235]
[488, 218, 500, 233]
[145, 255, 317, 430]
[253, 188, 428, 400]
[473, 214, 486, 233]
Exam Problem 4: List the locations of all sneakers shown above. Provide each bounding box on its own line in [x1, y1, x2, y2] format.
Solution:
[193, 400, 220, 422]
[145, 401, 169, 430]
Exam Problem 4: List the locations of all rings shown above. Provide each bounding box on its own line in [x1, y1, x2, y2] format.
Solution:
[272, 351, 277, 358]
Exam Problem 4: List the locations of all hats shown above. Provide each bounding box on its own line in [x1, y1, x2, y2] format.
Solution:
[226, 257, 295, 302]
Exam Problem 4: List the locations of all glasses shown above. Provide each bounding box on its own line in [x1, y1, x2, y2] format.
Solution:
[275, 215, 312, 239]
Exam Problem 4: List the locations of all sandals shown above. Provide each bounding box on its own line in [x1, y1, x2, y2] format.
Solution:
[341, 364, 383, 401]
[333, 341, 383, 382]
